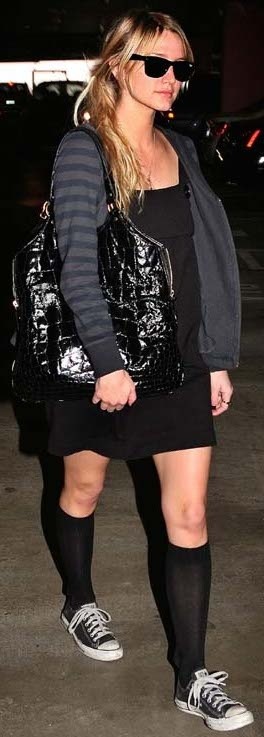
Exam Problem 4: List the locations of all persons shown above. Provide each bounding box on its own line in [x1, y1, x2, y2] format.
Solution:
[25, 6, 257, 737]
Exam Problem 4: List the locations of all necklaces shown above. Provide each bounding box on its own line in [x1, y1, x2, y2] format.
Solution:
[134, 130, 158, 191]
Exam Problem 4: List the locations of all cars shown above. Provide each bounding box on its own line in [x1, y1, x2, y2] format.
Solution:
[0, 81, 264, 192]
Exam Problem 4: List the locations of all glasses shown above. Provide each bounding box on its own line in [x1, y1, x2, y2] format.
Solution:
[131, 53, 196, 82]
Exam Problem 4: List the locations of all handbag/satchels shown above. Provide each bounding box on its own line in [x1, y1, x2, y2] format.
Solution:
[12, 126, 186, 403]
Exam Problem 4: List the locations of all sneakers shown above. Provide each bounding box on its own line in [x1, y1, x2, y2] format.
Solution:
[175, 669, 254, 731]
[59, 604, 124, 661]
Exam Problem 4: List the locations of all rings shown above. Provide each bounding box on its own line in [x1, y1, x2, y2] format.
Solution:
[222, 398, 232, 406]
